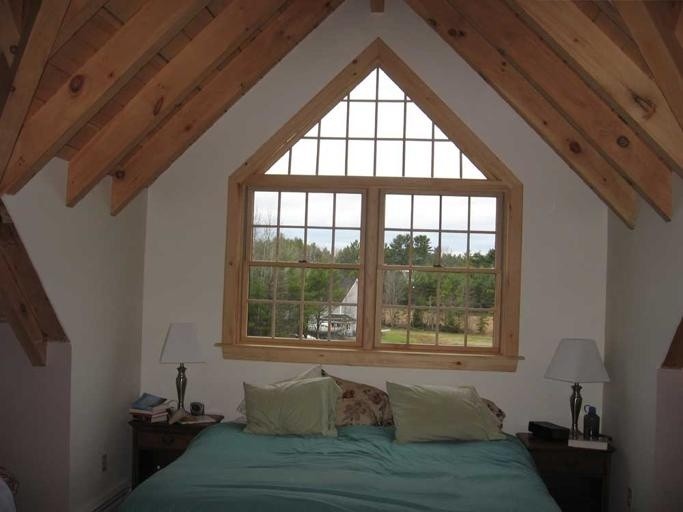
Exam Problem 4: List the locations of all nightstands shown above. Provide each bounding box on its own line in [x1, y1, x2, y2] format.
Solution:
[128, 415, 224, 492]
[516, 433, 619, 512]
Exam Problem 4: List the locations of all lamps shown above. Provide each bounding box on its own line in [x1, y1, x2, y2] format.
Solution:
[543, 339, 611, 439]
[160, 322, 210, 421]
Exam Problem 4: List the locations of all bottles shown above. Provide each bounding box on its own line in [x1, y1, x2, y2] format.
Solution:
[583, 405, 600, 434]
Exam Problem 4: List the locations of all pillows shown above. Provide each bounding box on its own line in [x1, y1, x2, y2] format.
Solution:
[219, 364, 506, 444]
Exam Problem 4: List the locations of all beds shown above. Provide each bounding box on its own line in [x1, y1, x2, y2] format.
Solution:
[116, 423, 560, 512]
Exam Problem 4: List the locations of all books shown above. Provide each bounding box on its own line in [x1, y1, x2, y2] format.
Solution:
[128, 392, 216, 424]
[568, 434, 608, 451]
